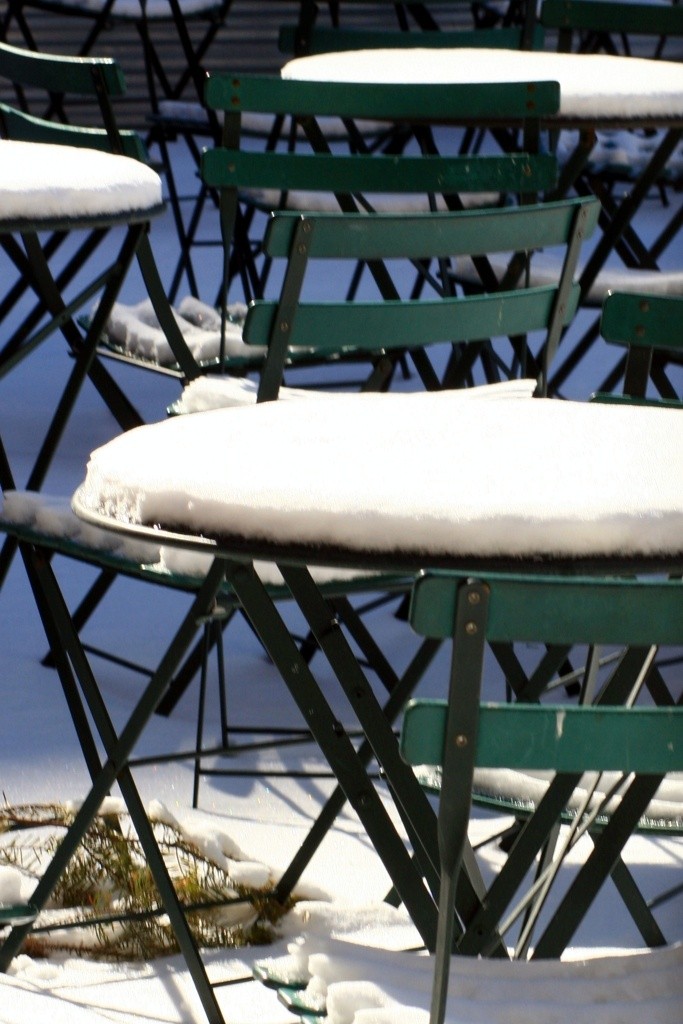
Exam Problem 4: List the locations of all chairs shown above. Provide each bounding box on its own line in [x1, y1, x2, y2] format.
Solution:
[0, 0, 683, 1024]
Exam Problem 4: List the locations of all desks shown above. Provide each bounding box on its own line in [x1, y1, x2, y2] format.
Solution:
[0, 199, 166, 585]
[295, 116, 683, 399]
[0, 483, 682, 1024]
